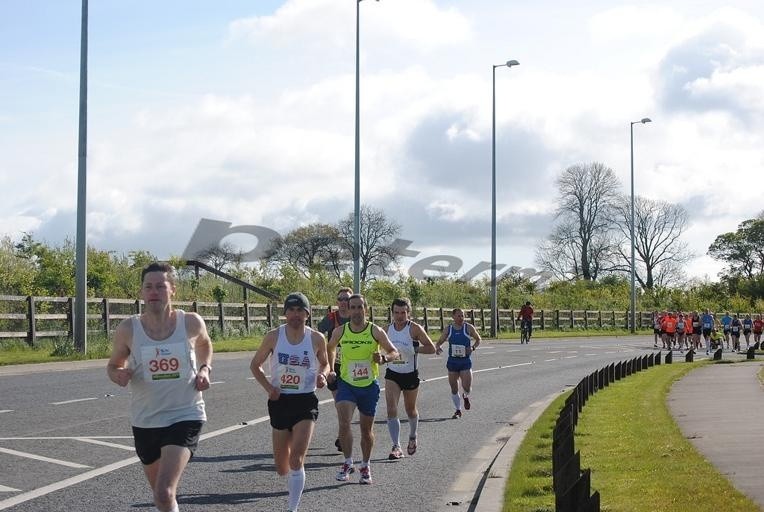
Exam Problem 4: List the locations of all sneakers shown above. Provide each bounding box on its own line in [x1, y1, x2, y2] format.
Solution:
[359, 467, 371, 484]
[407, 435, 417, 455]
[389, 446, 404, 458]
[453, 410, 463, 419]
[336, 465, 355, 481]
[463, 393, 470, 410]
[335, 439, 342, 452]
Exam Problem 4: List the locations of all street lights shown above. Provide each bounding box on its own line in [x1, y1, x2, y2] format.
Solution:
[631, 117, 652, 333]
[491, 60, 521, 340]
[353, 0, 381, 293]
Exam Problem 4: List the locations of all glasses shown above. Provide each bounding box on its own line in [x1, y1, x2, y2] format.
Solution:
[337, 297, 349, 302]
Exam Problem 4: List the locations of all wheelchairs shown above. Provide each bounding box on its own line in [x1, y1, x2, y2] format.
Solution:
[710, 338, 724, 350]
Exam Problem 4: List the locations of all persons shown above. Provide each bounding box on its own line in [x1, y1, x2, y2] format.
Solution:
[436, 309, 481, 418]
[326, 293, 398, 484]
[381, 297, 436, 459]
[517, 302, 534, 337]
[106, 263, 214, 512]
[652, 308, 764, 355]
[318, 287, 354, 452]
[249, 291, 331, 511]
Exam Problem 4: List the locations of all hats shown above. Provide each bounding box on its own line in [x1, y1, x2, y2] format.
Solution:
[284, 292, 310, 315]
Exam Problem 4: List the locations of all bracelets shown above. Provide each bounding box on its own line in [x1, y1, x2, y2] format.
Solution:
[382, 354, 388, 364]
[320, 371, 328, 381]
[472, 345, 475, 350]
[200, 363, 211, 375]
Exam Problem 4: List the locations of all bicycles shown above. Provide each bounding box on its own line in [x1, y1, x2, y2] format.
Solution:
[517, 319, 530, 344]
